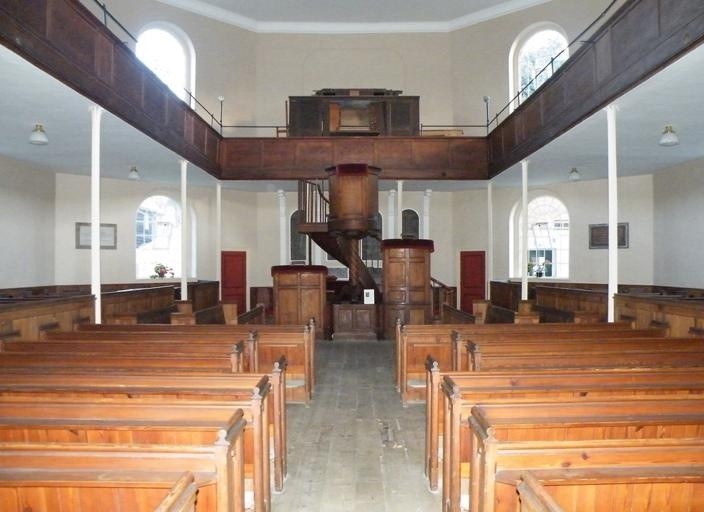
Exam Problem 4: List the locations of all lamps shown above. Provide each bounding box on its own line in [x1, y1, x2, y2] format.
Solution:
[29, 125, 49, 145]
[128, 167, 141, 181]
[658, 126, 679, 147]
[567, 168, 582, 181]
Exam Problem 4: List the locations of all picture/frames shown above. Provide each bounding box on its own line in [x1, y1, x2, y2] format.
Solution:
[75, 222, 117, 250]
[588, 222, 629, 249]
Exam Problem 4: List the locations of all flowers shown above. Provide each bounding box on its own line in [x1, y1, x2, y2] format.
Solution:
[149, 264, 175, 279]
